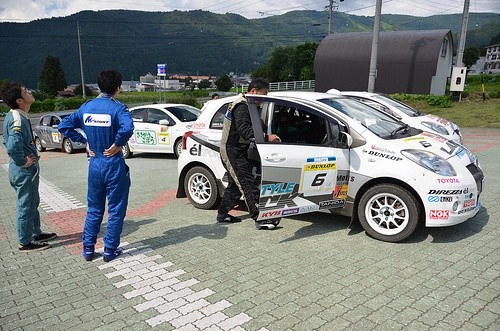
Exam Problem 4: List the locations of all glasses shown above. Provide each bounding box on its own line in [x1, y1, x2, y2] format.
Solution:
[20, 87, 29, 97]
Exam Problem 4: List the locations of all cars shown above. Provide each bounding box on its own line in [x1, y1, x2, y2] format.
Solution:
[327, 88, 463, 147]
[119, 103, 203, 159]
[32, 112, 89, 154]
[175, 89, 485, 245]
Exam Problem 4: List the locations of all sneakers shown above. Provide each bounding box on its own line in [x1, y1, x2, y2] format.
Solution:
[103, 246, 122, 262]
[217, 214, 241, 223]
[17, 240, 50, 252]
[34, 233, 57, 242]
[84, 246, 94, 261]
[254, 218, 281, 230]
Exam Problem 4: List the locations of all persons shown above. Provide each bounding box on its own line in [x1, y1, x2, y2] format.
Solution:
[216, 78, 281, 230]
[3, 84, 57, 252]
[58, 71, 134, 262]
